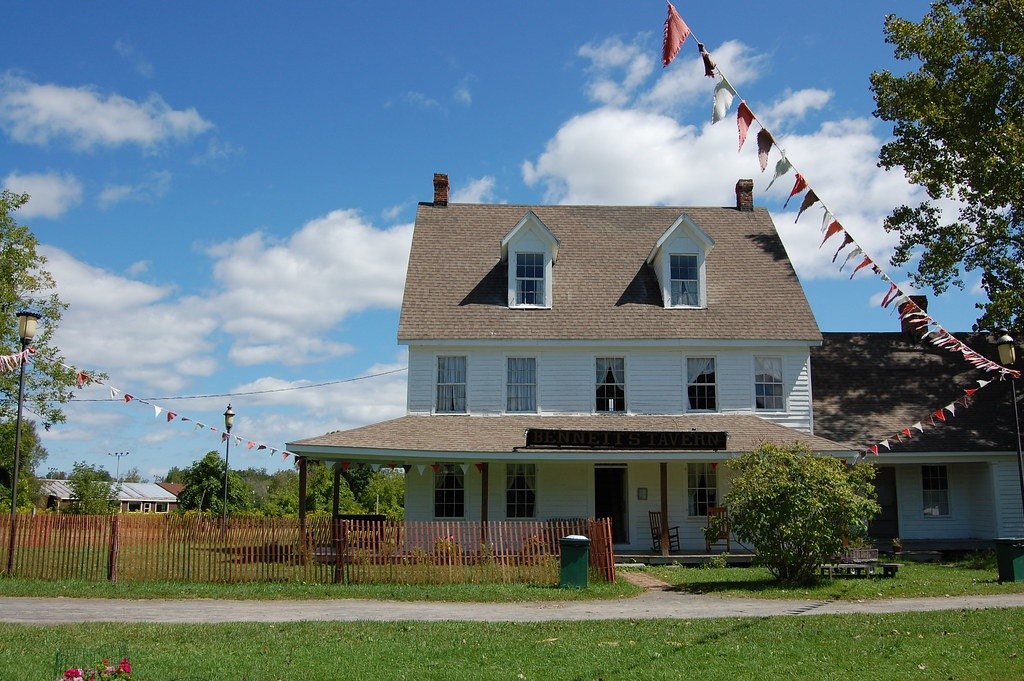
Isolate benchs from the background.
[793,564,904,579]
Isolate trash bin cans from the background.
[558,535,591,588]
[992,537,1024,581]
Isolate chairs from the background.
[704,506,730,553]
[648,510,682,555]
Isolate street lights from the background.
[6,301,42,575]
[223,403,234,539]
[109,451,130,482]
[995,327,1023,508]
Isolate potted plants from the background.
[892,538,903,553]
